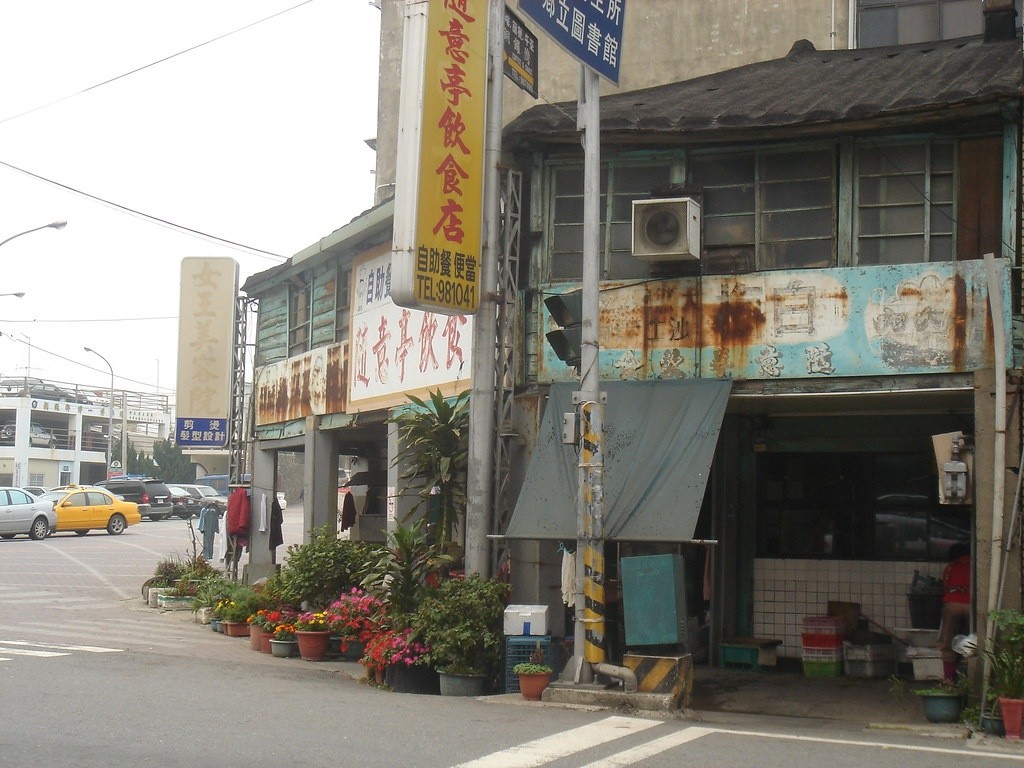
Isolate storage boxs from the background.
[506,635,559,694]
[504,604,550,635]
[801,594,950,680]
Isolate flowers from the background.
[245,588,431,670]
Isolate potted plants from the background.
[918,686,962,723]
[419,571,510,698]
[981,711,1003,736]
[962,637,1024,740]
[514,663,552,701]
[142,524,251,637]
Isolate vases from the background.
[249,624,426,694]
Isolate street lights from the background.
[83,346,113,481]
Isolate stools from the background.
[723,638,782,674]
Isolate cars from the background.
[18,383,110,405]
[0,484,140,541]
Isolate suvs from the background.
[95,477,228,521]
[0,420,57,449]
